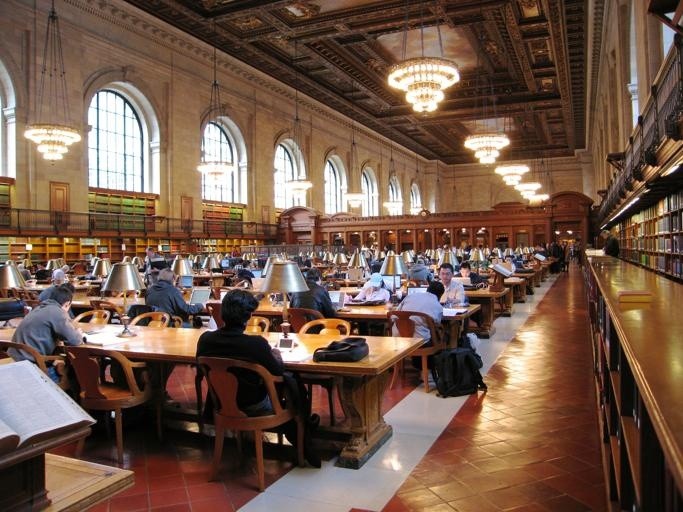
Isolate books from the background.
[0,360,96,458]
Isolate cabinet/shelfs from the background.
[581,257,683,512]
[612,192,683,280]
[1,236,264,263]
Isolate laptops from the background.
[327,291,345,311]
[250,270,262,278]
[219,259,229,270]
[189,288,211,309]
[177,276,192,292]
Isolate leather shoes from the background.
[292,412,320,447]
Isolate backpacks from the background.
[431,347,488,396]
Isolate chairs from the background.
[1,247,535,491]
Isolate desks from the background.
[41,452,135,512]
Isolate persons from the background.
[290,270,336,334]
[392,282,445,382]
[439,263,465,342]
[4,261,74,318]
[7,286,83,382]
[196,289,320,445]
[302,256,311,268]
[356,252,435,336]
[238,260,255,285]
[546,241,577,274]
[144,248,206,328]
[456,244,546,326]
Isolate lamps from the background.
[382,72,402,215]
[387,1,460,117]
[408,138,424,214]
[340,56,369,211]
[465,47,550,206]
[278,36,317,204]
[196,15,242,189]
[23,1,82,164]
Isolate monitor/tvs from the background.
[380,275,394,294]
[394,275,401,289]
[408,287,427,296]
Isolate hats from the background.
[368,272,383,287]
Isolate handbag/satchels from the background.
[312,338,369,362]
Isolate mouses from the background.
[341,308,351,312]
